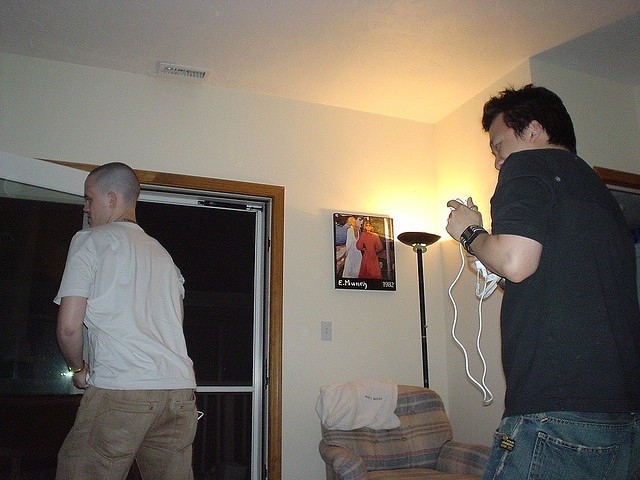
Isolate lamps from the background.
[396,230,443,393]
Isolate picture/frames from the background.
[331,213,397,292]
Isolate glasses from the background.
[366,225,371,227]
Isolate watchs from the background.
[459,226,489,255]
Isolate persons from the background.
[447,84,636,479]
[54,162,198,479]
[342,217,384,279]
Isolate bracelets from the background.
[67,360,86,373]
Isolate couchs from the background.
[314,385,492,479]
